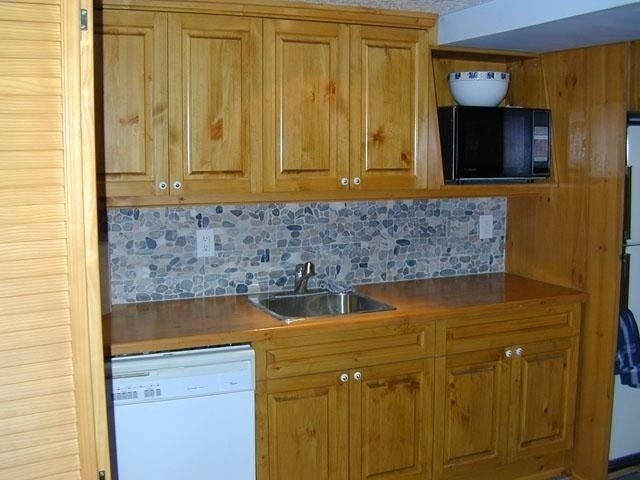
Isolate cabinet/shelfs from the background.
[0,1,113,479]
[254,310,438,480]
[439,293,588,480]
[259,1,439,201]
[95,2,262,206]
[427,46,558,198]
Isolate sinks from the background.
[248,288,397,324]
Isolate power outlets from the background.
[479,215,491,236]
[196,230,214,256]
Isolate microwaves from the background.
[437,105,555,185]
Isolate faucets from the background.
[294,261,316,292]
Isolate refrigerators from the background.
[603,117,640,474]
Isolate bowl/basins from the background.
[448,69,512,108]
